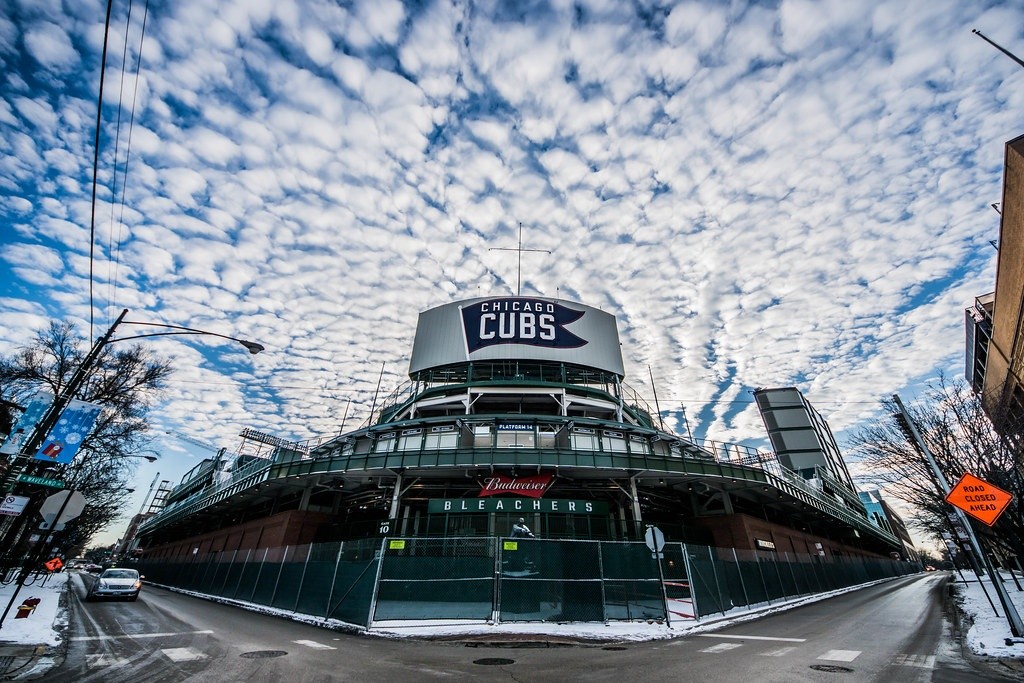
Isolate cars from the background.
[68,563,102,573]
[90,569,146,602]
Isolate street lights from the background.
[0,309,264,506]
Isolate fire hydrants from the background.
[14,598,41,619]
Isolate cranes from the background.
[165,430,237,460]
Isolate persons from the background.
[510,517,535,568]
